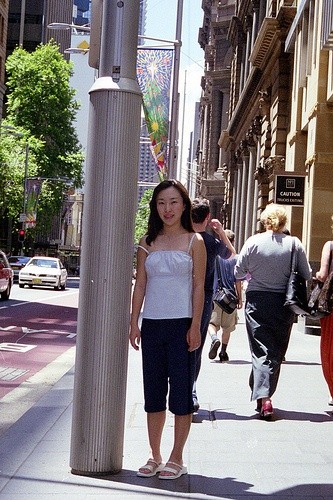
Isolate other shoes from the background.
[193,398,200,411]
[208,339,221,359]
[257,399,262,411]
[219,353,229,362]
[328,401,333,405]
[260,404,274,417]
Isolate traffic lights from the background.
[18,229,25,242]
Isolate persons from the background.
[315,241,333,405]
[233,203,312,418]
[129,179,206,480]
[208,229,243,362]
[191,204,236,411]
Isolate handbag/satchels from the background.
[307,241,333,315]
[213,288,239,314]
[283,237,320,321]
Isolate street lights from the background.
[46,0,185,180]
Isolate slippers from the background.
[136,459,165,477]
[159,462,188,479]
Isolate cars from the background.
[7,256,32,284]
[18,256,68,291]
[0,250,14,301]
